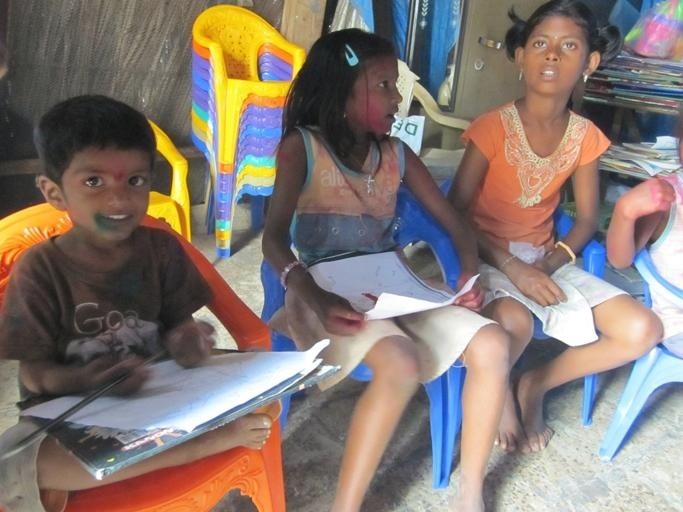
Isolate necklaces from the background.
[348,140,381,198]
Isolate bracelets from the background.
[499,255,517,271]
[280,261,308,288]
[553,241,576,266]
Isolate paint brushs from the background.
[0,349,167,460]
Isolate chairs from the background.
[432,177,605,426]
[398,73,473,180]
[140,116,193,239]
[1,201,288,510]
[187,3,306,257]
[596,246,682,462]
[256,183,455,491]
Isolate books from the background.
[581,51,682,181]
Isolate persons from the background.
[0,93,273,512]
[606,111,683,359]
[259,27,513,511]
[446,1,664,455]
